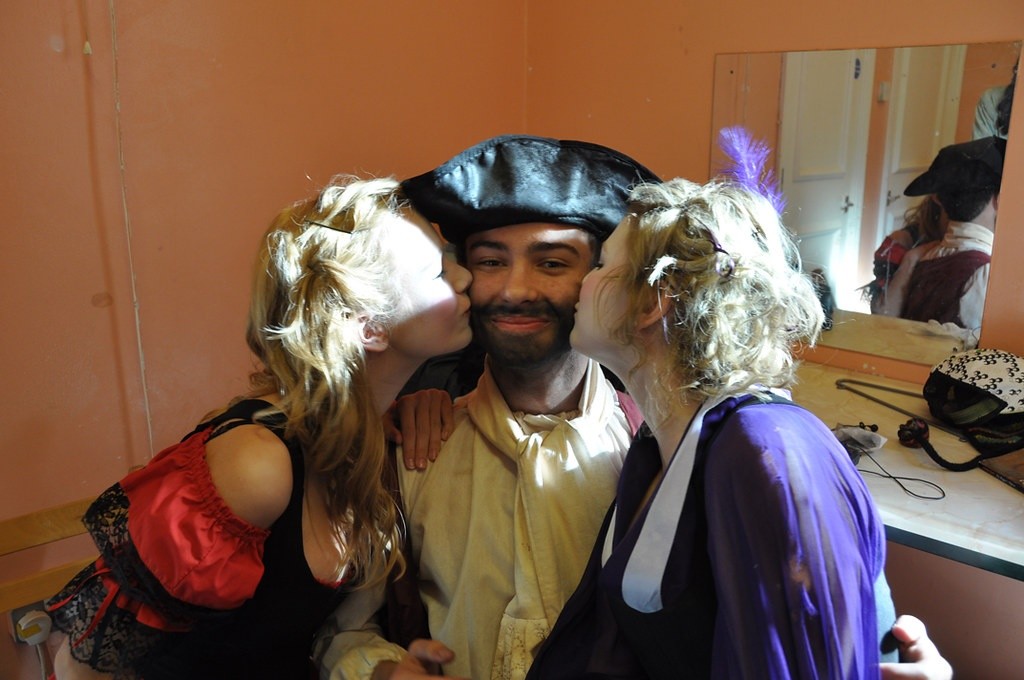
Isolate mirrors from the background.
[710,41,1023,367]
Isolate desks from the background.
[795,359,1024,680]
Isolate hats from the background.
[400,136,663,245]
[903,136,1008,198]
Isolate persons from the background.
[863,58,1019,329]
[50,127,957,680]
